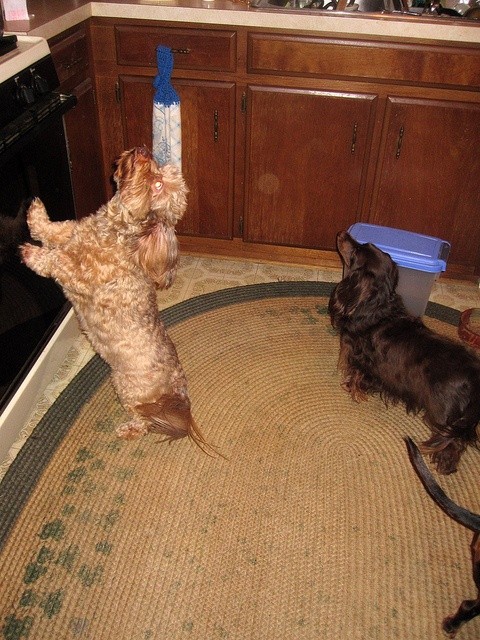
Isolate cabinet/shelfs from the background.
[50,30,108,220]
[109,26,236,242]
[243,33,479,282]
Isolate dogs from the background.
[327,230,479,475]
[17,147,225,462]
[398,434,480,636]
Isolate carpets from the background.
[0,282,480,636]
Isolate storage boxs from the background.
[345,222,450,319]
